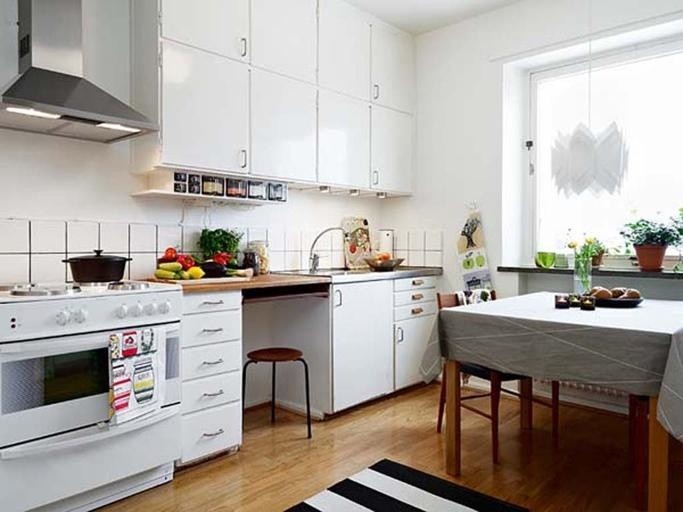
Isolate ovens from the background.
[0,292,181,511]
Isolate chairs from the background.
[436,291,560,464]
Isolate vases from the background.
[573,257,592,295]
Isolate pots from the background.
[61,251,132,282]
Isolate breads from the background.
[590,286,640,299]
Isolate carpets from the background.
[283,459,532,512]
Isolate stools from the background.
[242,347,312,439]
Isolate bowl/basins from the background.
[534,252,556,268]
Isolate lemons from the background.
[189,267,205,278]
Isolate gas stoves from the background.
[0,279,182,305]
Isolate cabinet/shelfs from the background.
[130,1,318,83]
[394,276,440,392]
[130,41,317,182]
[332,280,394,413]
[317,1,416,116]
[180,293,242,463]
[316,89,413,199]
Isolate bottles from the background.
[251,240,269,274]
[243,249,258,275]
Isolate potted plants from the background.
[620,219,682,271]
[582,238,605,266]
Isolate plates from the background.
[593,298,643,307]
[364,259,404,270]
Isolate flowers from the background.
[567,228,605,292]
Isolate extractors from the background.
[0,1,159,144]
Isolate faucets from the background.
[308,227,349,272]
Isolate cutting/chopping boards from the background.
[343,217,371,270]
[147,277,250,285]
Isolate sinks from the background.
[270,269,354,276]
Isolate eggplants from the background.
[198,262,253,278]
[154,262,190,279]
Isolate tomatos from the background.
[165,248,200,266]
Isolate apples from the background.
[377,253,391,260]
